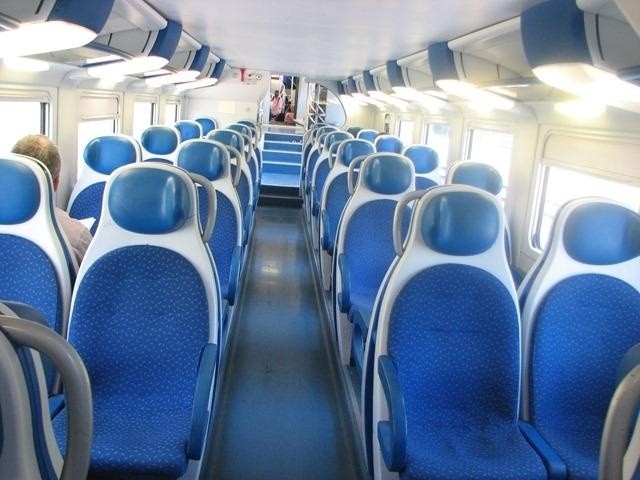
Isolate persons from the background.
[11,135,92,263]
[270,90,283,116]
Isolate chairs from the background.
[373,135,404,156]
[327,153,378,341]
[140,126,180,167]
[351,184,464,476]
[237,121,262,189]
[1,333,50,480]
[355,128,380,142]
[66,132,143,238]
[598,344,640,480]
[362,182,568,480]
[0,301,94,480]
[348,127,362,138]
[299,128,318,196]
[403,144,442,190]
[446,162,526,311]
[47,162,222,478]
[167,162,229,430]
[205,129,253,247]
[229,126,259,214]
[197,119,217,139]
[320,139,380,292]
[301,123,328,145]
[517,196,603,313]
[175,121,201,141]
[175,138,243,351]
[18,155,78,289]
[0,153,71,420]
[333,152,416,378]
[305,126,339,223]
[307,131,355,251]
[519,197,640,480]
